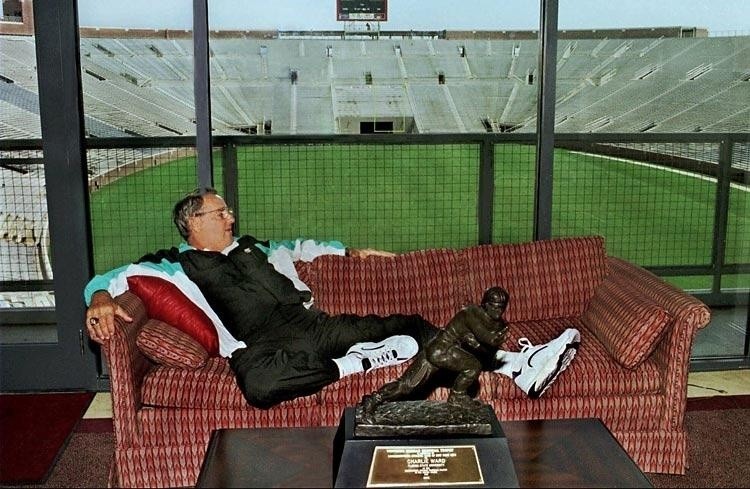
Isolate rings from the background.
[90,317,100,326]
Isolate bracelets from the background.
[345,247,350,257]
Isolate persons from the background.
[82,185,583,412]
[353,286,513,424]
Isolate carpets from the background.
[37,391,750,489]
[0,390,95,489]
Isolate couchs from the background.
[100,231,713,489]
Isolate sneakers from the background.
[509,328,581,400]
[345,334,419,374]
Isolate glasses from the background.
[195,206,234,219]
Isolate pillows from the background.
[580,268,678,374]
[133,313,215,374]
[125,272,226,357]
